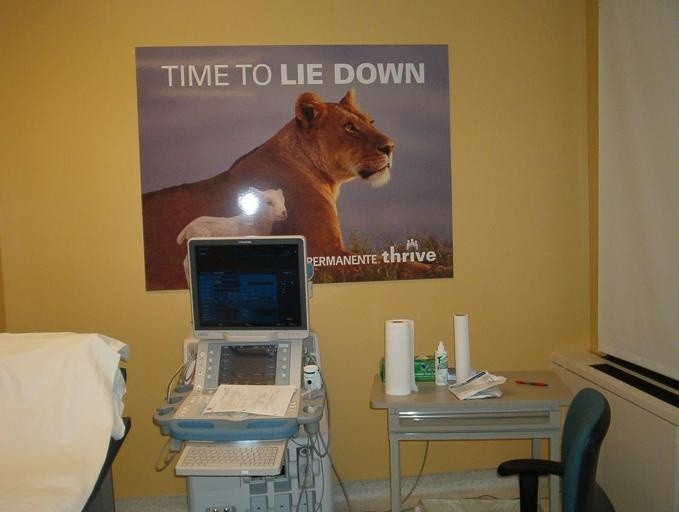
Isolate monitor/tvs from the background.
[187,237,309,339]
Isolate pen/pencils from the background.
[515,381,547,386]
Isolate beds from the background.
[1,330,132,511]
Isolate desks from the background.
[370,371,574,511]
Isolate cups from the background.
[303,365,323,391]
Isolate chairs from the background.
[414,384,614,511]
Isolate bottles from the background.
[435,341,449,386]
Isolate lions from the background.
[141,88,452,291]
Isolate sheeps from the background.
[176,186,287,288]
